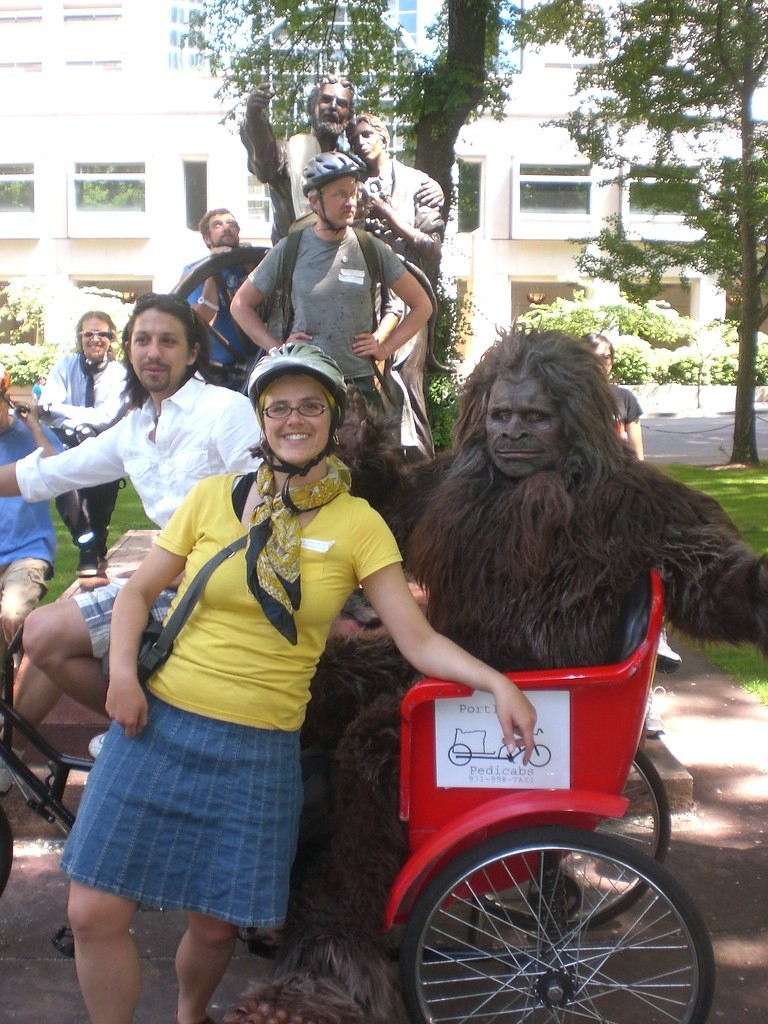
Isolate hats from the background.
[36,376,41,381]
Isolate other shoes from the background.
[75,548,99,576]
[0,767,14,795]
[87,731,109,759]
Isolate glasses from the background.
[135,292,195,324]
[262,401,327,419]
[328,191,357,200]
[78,331,108,337]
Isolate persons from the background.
[0,293,537,1024]
[230,152,433,410]
[580,332,644,461]
[239,76,457,466]
[178,207,273,367]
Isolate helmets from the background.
[247,341,348,410]
[0,365,11,395]
[301,151,360,198]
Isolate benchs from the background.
[348,568,663,933]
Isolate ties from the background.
[79,350,110,408]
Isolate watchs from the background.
[198,297,219,311]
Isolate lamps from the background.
[118,285,136,304]
[524,286,549,305]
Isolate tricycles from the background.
[0,566,715,1024]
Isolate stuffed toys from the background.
[237,322,768,1024]
